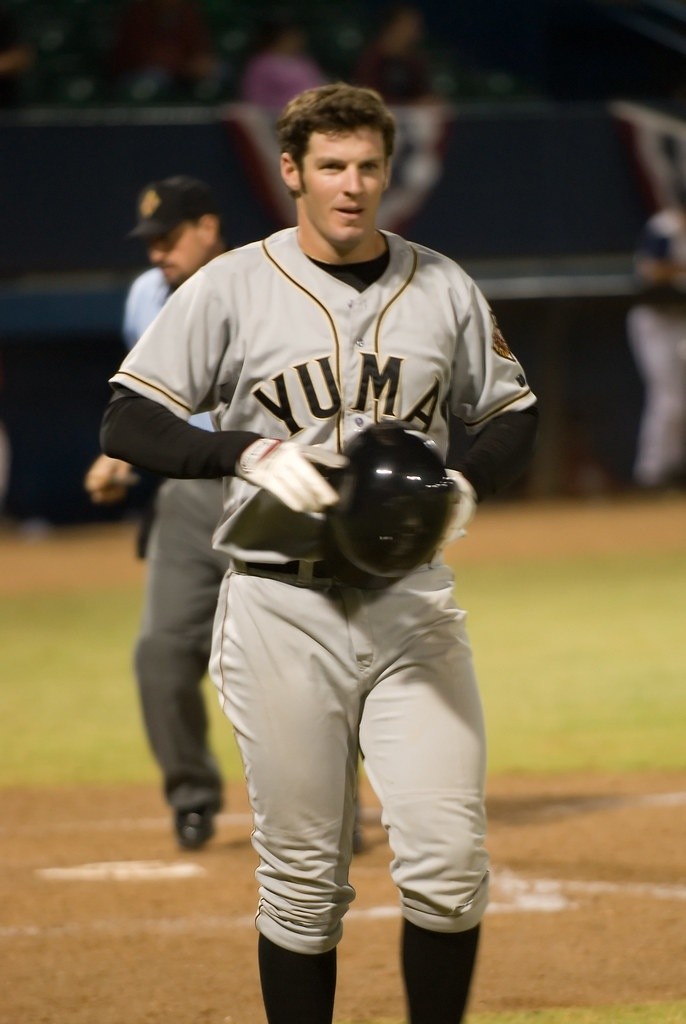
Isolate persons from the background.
[101,84,536,1024]
[80,179,235,845]
[623,186,686,487]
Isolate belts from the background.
[230,560,331,585]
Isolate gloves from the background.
[441,468,477,546]
[235,437,350,518]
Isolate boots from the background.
[258,932,337,1024]
[400,919,480,1024]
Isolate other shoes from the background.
[176,809,213,847]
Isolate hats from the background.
[124,171,220,237]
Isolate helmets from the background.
[328,418,456,579]
